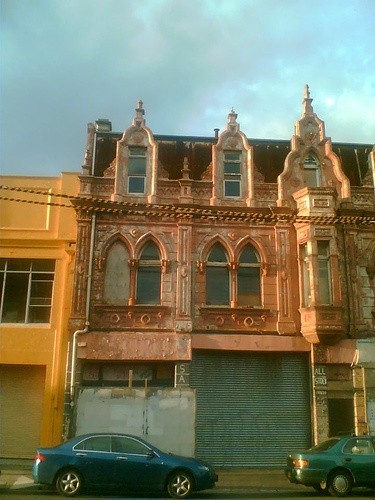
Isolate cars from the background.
[32,433,217,499]
[283,434,375,497]
